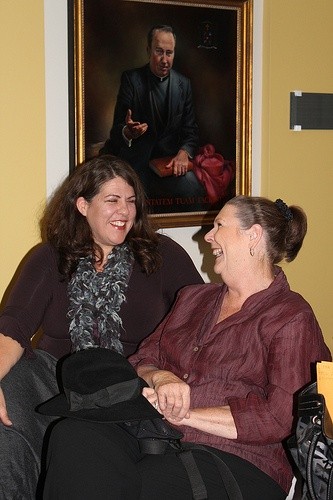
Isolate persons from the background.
[0,157,205,500]
[41,195,333,500]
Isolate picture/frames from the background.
[67,0,253,228]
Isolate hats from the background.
[33,347,165,424]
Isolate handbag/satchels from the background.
[280,380,333,500]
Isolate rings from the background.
[153,400,158,409]
